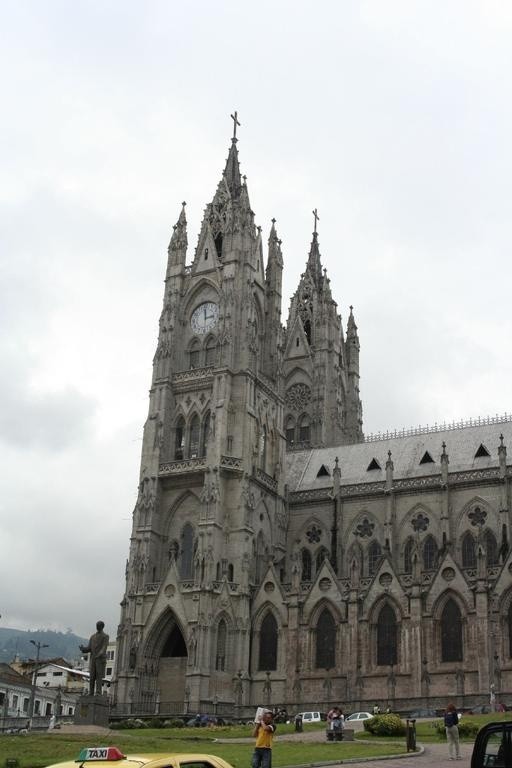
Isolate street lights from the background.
[25,638,51,718]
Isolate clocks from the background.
[188,300,221,336]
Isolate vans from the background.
[285,710,327,725]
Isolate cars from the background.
[343,712,375,721]
[460,703,509,715]
[40,744,235,768]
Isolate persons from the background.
[332,710,345,742]
[202,712,212,728]
[194,711,201,728]
[249,712,278,768]
[327,707,337,741]
[444,704,465,760]
[386,704,392,714]
[79,620,110,697]
[372,704,380,714]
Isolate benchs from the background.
[326,728,354,740]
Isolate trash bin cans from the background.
[407,719,416,752]
[7,757,19,768]
[296,716,303,733]
